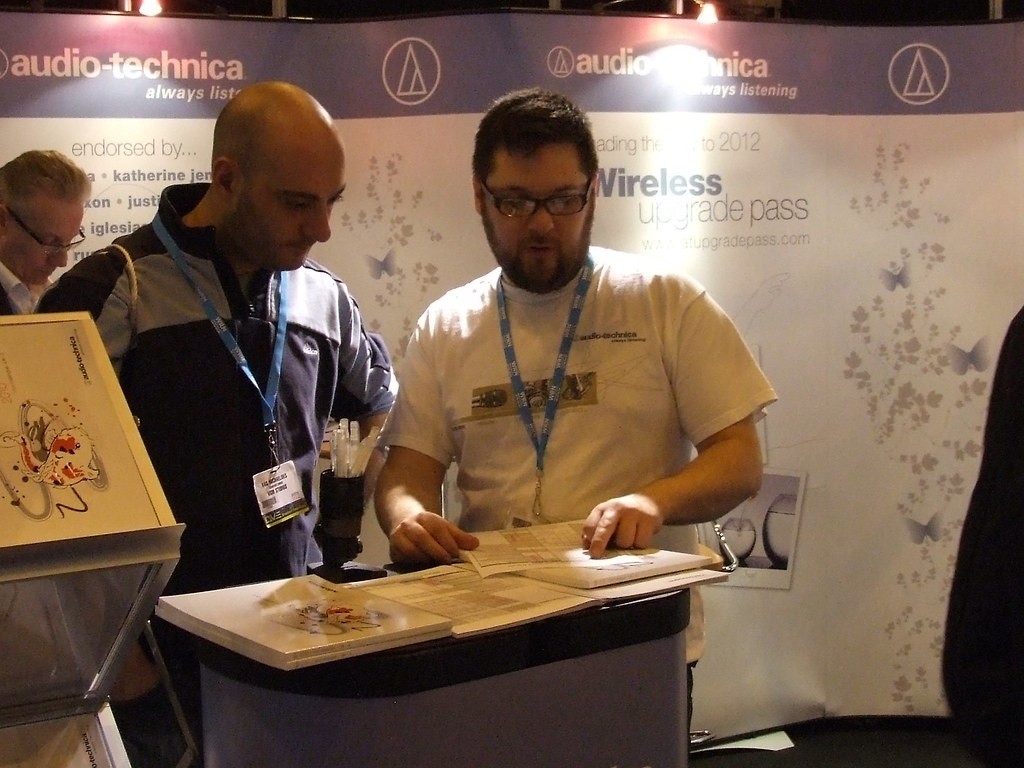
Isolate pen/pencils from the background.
[330,418,380,478]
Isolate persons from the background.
[943,305,1024,768]
[0,150,90,317]
[374,89,777,752]
[33,79,400,768]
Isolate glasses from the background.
[4,205,85,257]
[477,176,595,219]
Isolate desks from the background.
[152,566,726,768]
